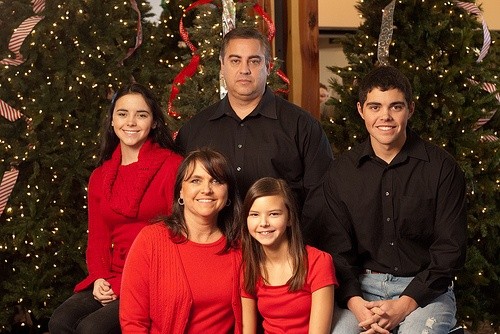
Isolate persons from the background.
[49,83,184,334]
[172,26,334,224]
[322,66,468,334]
[240,178,339,334]
[119,148,242,334]
